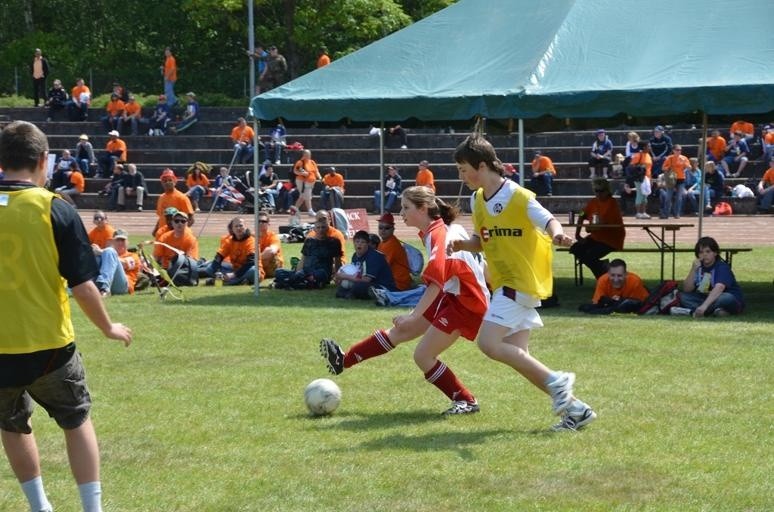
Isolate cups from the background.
[591,214,599,223]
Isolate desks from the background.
[561,224,695,284]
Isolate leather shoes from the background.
[100,291,111,296]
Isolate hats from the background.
[160,170,177,180]
[80,135,88,140]
[164,207,188,218]
[113,230,127,240]
[377,214,394,224]
[108,129,119,136]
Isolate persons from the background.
[232,116,288,165]
[415,159,436,195]
[0,119,133,512]
[530,148,558,198]
[369,122,408,148]
[33,46,90,117]
[446,131,598,434]
[248,46,331,97]
[589,258,650,313]
[677,236,745,319]
[162,47,179,108]
[568,120,774,219]
[48,132,149,213]
[568,177,626,281]
[88,210,412,299]
[319,185,494,415]
[152,172,195,235]
[374,166,404,213]
[182,148,345,217]
[107,83,199,136]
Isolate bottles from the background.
[214,271,223,288]
[287,156,290,163]
[671,306,696,317]
[568,210,575,224]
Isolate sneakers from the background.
[715,309,731,316]
[442,400,480,415]
[117,206,143,211]
[309,209,317,217]
[547,373,597,431]
[320,339,344,374]
[636,212,651,219]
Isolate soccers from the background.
[304,379,341,414]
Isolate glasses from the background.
[379,226,392,229]
[175,220,186,224]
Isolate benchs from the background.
[556,248,752,288]
